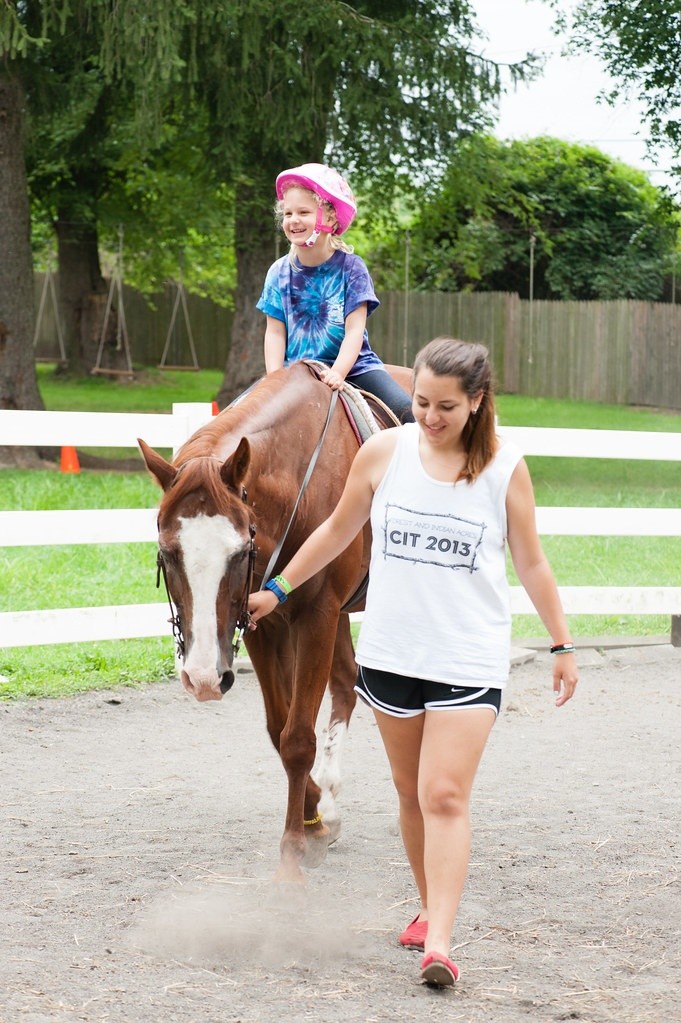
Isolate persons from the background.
[256,163,417,426]
[249,337,579,986]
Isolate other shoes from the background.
[420,953,458,984]
[400,912,429,951]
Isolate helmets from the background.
[276,162,358,235]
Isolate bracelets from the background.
[263,578,287,604]
[550,641,576,654]
[275,575,292,593]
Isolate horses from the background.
[136,359,416,885]
